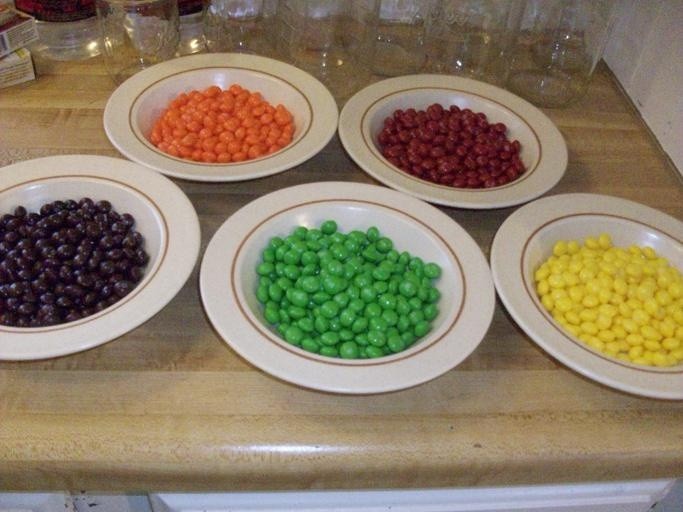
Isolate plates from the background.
[2,151,202,364]
[339,72,569,209]
[489,190,680,402]
[206,180,498,397]
[101,52,340,183]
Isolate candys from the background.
[378,102,526,188]
[536,235,682,365]
[255,220,442,359]
[150,83,295,164]
[0,197,151,327]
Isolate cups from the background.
[95,1,623,108]
